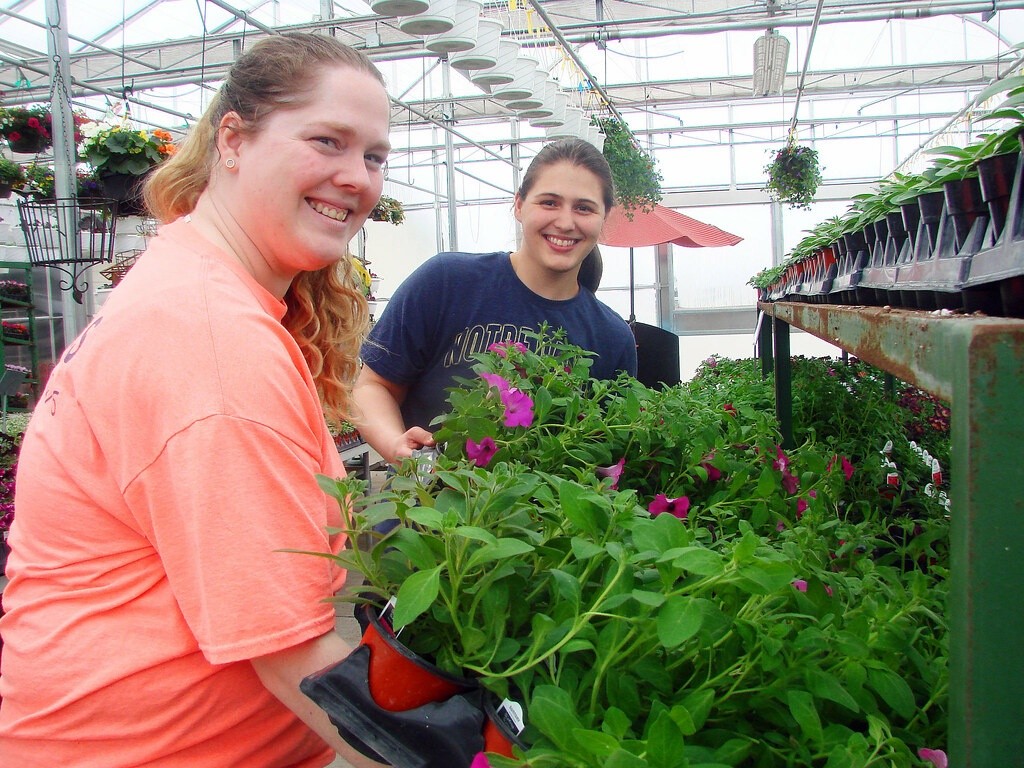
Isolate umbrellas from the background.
[598,190,742,248]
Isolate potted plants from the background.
[746,41,1024,303]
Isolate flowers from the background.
[12,162,53,196]
[0,279,29,294]
[5,364,31,374]
[0,106,88,156]
[76,168,97,194]
[2,321,30,334]
[9,392,31,403]
[596,112,664,222]
[0,410,34,542]
[760,143,826,211]
[81,126,177,175]
[0,158,27,184]
[275,321,1024,768]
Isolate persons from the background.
[1,29,398,768]
[353,138,640,477]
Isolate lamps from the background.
[752,30,791,98]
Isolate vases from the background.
[580,117,594,142]
[3,333,29,346]
[1,295,28,310]
[506,70,549,109]
[397,0,457,35]
[7,133,42,153]
[545,108,584,141]
[517,81,558,119]
[424,0,483,53]
[359,602,461,712]
[35,192,56,204]
[0,180,12,198]
[8,401,27,407]
[0,544,8,573]
[101,174,148,218]
[530,92,567,128]
[492,56,539,99]
[77,192,103,210]
[595,134,607,153]
[368,0,430,16]
[447,17,504,70]
[588,126,599,145]
